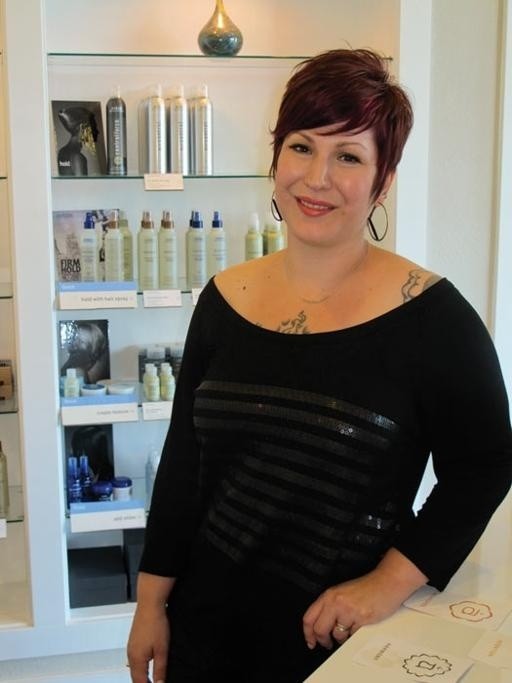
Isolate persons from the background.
[123,47,511,682]
[57,106,100,177]
[61,322,108,384]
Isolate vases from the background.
[196,0,242,56]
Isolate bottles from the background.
[144,448,161,498]
[97,475,133,503]
[65,455,94,510]
[65,367,81,403]
[140,340,184,402]
[142,83,216,177]
[80,211,227,289]
[242,209,284,265]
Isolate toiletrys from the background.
[80,212,98,282]
[185,212,206,290]
[106,211,134,283]
[206,209,226,277]
[246,213,283,258]
[148,83,211,175]
[159,210,178,291]
[65,455,134,504]
[136,211,157,290]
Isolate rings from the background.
[335,621,349,631]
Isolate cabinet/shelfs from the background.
[0,174,36,631]
[50,47,432,613]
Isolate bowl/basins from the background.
[81,379,138,396]
